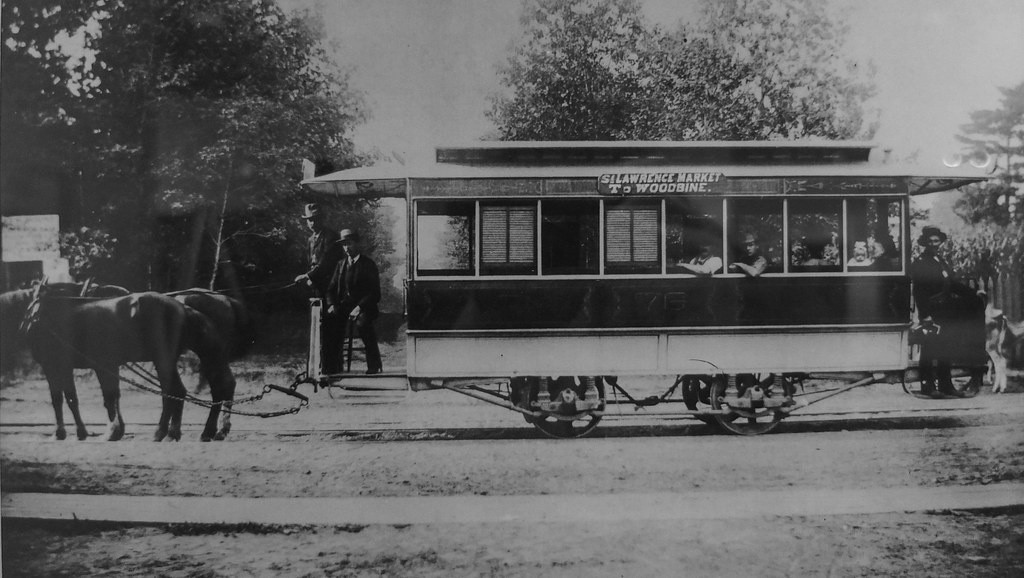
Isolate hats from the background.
[301,204,322,219]
[917,225,946,246]
[336,229,359,243]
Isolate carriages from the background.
[0,136,1000,442]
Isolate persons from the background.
[294,201,341,298]
[675,238,723,274]
[913,223,960,401]
[729,232,767,277]
[849,241,873,266]
[326,230,383,375]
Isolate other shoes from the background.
[366,365,383,374]
[922,389,945,399]
[940,387,965,397]
[322,365,343,375]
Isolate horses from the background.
[0,276,253,442]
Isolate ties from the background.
[351,258,354,265]
